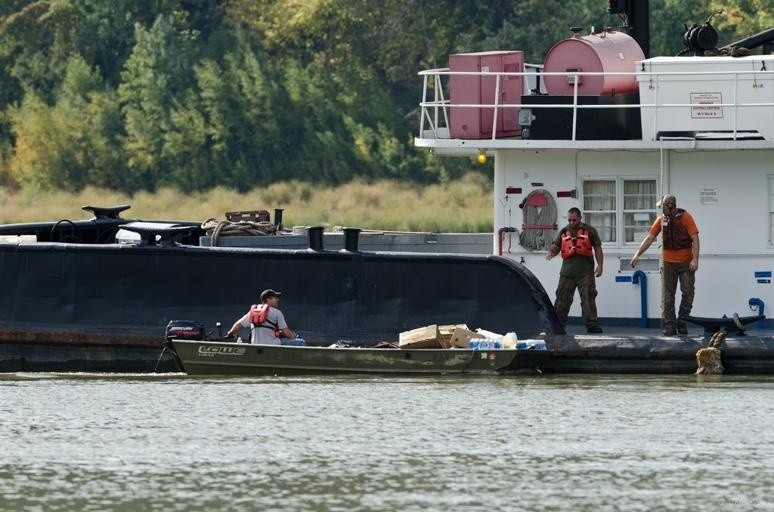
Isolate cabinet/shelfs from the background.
[448,50,524,139]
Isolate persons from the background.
[547,207,603,333]
[226,289,305,345]
[631,194,699,336]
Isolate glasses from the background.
[569,219,576,221]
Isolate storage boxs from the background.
[398,323,486,349]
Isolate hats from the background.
[260,289,282,300]
[656,194,676,208]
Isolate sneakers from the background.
[587,325,602,333]
[664,321,688,335]
